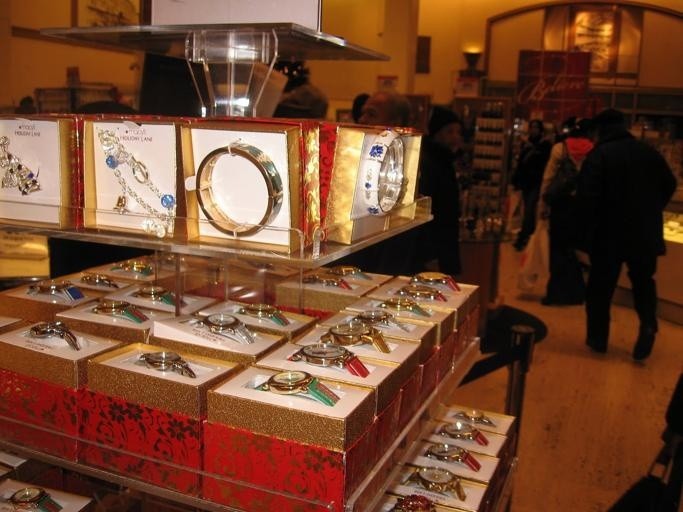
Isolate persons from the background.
[273,84,462,277]
[508,108,676,360]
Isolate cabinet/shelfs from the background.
[0,0,519,512]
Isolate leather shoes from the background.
[584,338,609,353]
[632,324,656,361]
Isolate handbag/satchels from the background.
[511,220,551,300]
[605,477,666,511]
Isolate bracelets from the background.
[198,143,283,237]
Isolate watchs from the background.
[1,254,497,511]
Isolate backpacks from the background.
[543,142,580,219]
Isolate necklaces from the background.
[0,130,175,227]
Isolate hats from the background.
[427,104,461,135]
